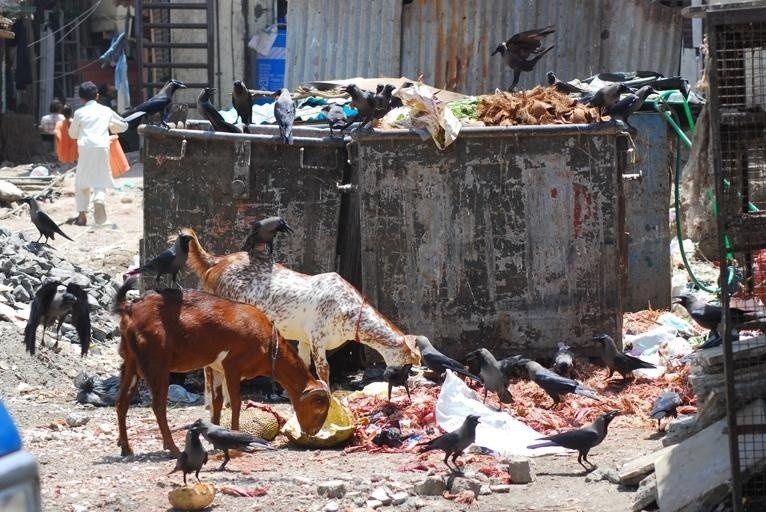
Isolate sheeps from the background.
[111,275,332,459]
[167,227,423,421]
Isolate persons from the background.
[41,98,67,135]
[64,104,74,128]
[68,80,129,227]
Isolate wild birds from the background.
[180,418,278,472]
[646,391,682,433]
[717,264,739,297]
[130,233,196,289]
[16,196,75,244]
[239,215,294,261]
[526,409,621,472]
[414,413,483,475]
[23,279,91,358]
[490,25,557,93]
[545,69,660,130]
[382,333,658,412]
[167,426,209,486]
[119,77,414,146]
[670,292,766,339]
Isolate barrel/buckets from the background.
[111,133,130,179]
[257,19,286,104]
